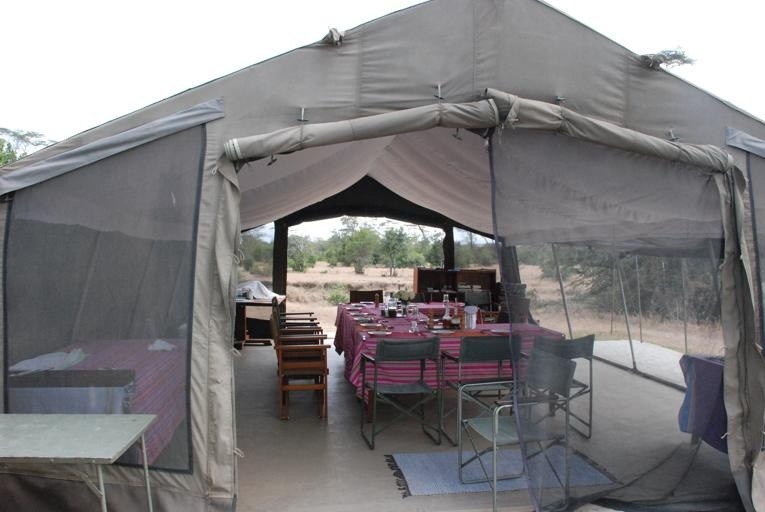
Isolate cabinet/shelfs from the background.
[414,267,498,295]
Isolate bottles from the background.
[441,298,461,330]
[380,293,404,317]
[237,288,254,300]
[375,294,379,308]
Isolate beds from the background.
[8,335,188,467]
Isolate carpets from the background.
[382,440,625,499]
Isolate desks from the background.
[0,412,162,512]
[234,294,288,350]
[677,351,727,456]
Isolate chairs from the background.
[436,336,531,448]
[359,336,442,451]
[269,288,532,420]
[457,348,577,512]
[517,333,597,439]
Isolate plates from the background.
[346,300,374,317]
[355,317,392,337]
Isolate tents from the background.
[0,0,765,512]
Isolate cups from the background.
[443,294,449,305]
[464,307,478,330]
[409,322,417,333]
[406,303,420,320]
